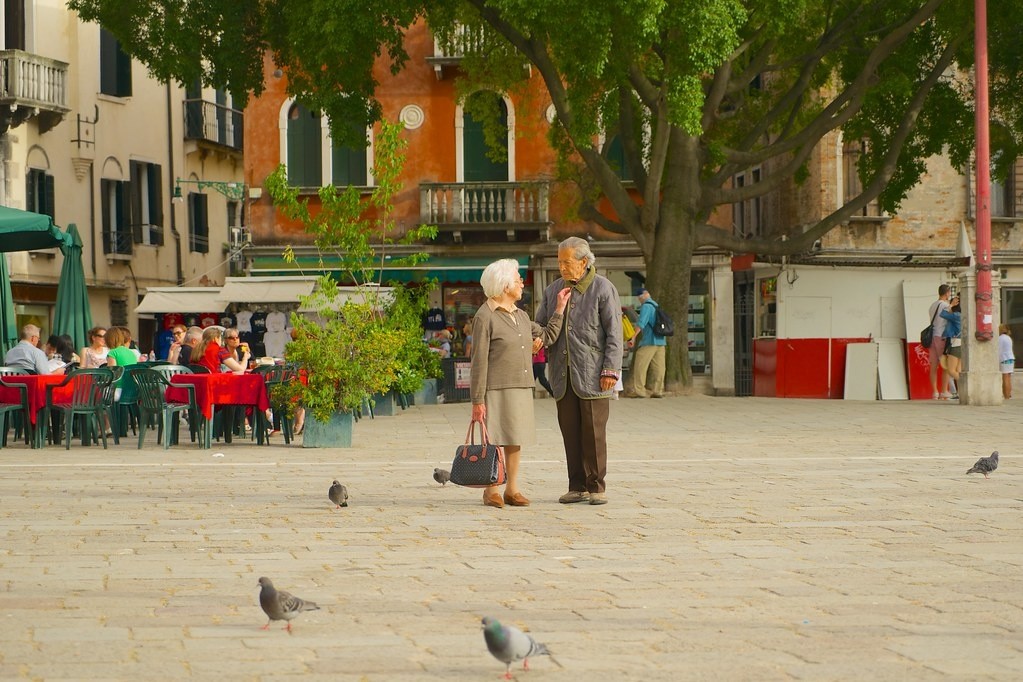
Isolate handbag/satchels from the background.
[449,419,507,488]
[921,325,933,348]
[943,337,952,355]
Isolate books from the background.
[48,358,80,373]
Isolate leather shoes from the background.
[483,490,504,507]
[503,490,531,506]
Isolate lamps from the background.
[171,177,246,203]
[451,288,460,295]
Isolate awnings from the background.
[134,292,230,313]
[216,282,316,303]
[296,291,397,313]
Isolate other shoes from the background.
[932,392,939,400]
[627,391,645,398]
[948,393,959,400]
[650,393,662,398]
[609,393,619,400]
[939,392,953,401]
[559,491,590,503]
[590,493,608,505]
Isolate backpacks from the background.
[642,302,674,337]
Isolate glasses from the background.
[32,335,41,341]
[225,337,238,340]
[173,331,185,335]
[514,278,523,284]
[94,334,104,338]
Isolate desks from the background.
[225,368,339,432]
[0,375,102,446]
[164,374,270,450]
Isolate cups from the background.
[249,360,256,369]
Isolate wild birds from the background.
[253,577,322,632]
[433,467,451,488]
[480,615,549,679]
[328,480,350,509]
[965,451,1000,479]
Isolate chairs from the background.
[0,361,412,449]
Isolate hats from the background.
[637,288,647,295]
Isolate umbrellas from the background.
[0,205,94,366]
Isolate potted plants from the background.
[262,120,446,449]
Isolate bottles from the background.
[149,348,156,362]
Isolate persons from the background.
[158,325,297,437]
[532,237,624,505]
[463,323,473,358]
[621,289,667,398]
[610,307,635,400]
[998,324,1015,400]
[532,345,554,399]
[80,326,142,439]
[471,258,572,508]
[4,324,67,375]
[292,328,314,436]
[44,334,80,439]
[928,285,962,401]
[436,330,450,359]
